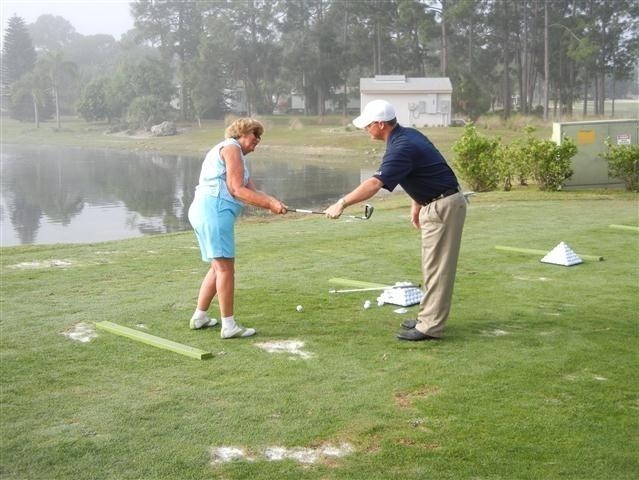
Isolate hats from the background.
[353,101,396,128]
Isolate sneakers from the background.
[220,323,254,339]
[189,317,216,329]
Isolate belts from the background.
[422,188,458,206]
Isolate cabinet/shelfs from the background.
[548,118,639,191]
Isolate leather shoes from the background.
[398,329,434,340]
[402,319,418,328]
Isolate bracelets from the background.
[338,198,346,207]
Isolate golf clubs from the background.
[285,205,374,220]
[328,285,421,293]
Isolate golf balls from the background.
[376,281,423,306]
[364,301,371,309]
[541,242,582,265]
[296,306,303,311]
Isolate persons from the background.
[324,99,467,340]
[185,117,290,339]
